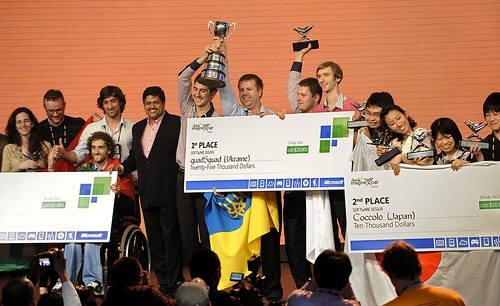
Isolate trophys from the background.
[292,25,320,51]
[366,129,402,167]
[346,100,369,129]
[406,130,434,160]
[198,21,236,88]
[460,120,489,149]
[21,151,42,172]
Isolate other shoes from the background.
[52,279,63,294]
[87,281,105,298]
[39,286,49,295]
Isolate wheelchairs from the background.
[101,194,151,279]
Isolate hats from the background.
[174,281,209,306]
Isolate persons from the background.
[0,106,54,260]
[107,86,184,298]
[351,90,500,176]
[286,249,361,306]
[175,44,219,249]
[0,249,82,306]
[214,36,283,306]
[282,42,361,291]
[381,241,466,306]
[51,86,141,296]
[37,89,102,149]
[99,246,239,306]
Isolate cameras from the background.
[32,251,55,271]
[230,272,244,282]
